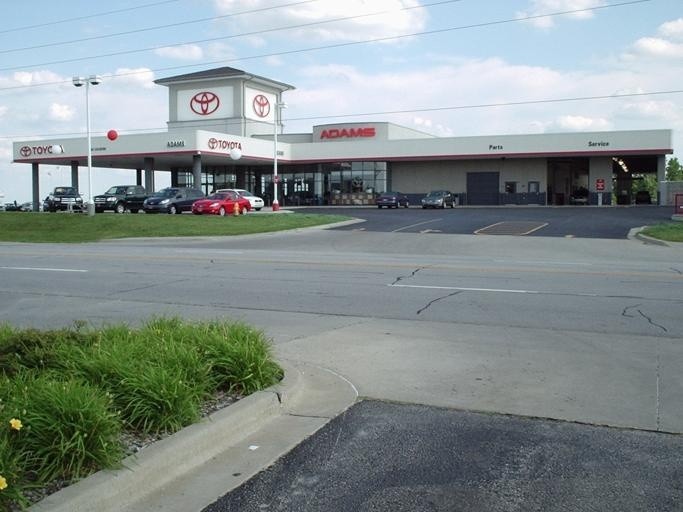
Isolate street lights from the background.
[272,101,288,211]
[73,75,102,216]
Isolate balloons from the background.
[51,146,62,156]
[107,130,117,141]
[229,147,242,161]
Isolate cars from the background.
[93,185,264,216]
[636,191,651,204]
[421,191,456,209]
[570,191,588,205]
[43,187,86,213]
[376,192,409,209]
